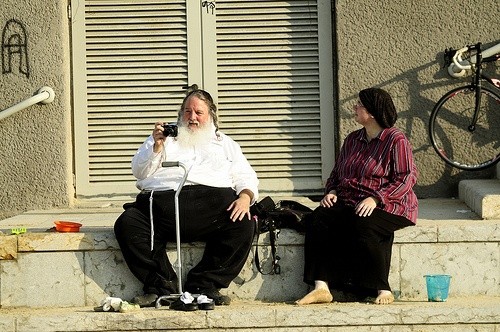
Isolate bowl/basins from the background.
[54,221,82,231]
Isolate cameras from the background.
[160,122,178,137]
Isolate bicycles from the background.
[428,40,499,172]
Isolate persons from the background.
[294,88,419,305]
[114,89,260,308]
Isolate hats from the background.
[359,88,398,130]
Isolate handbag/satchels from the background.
[250,196,281,275]
[261,199,313,235]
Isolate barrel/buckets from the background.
[423,274,452,302]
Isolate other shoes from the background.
[130,293,158,306]
[205,290,231,306]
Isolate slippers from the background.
[170,296,198,311]
[197,303,215,310]
